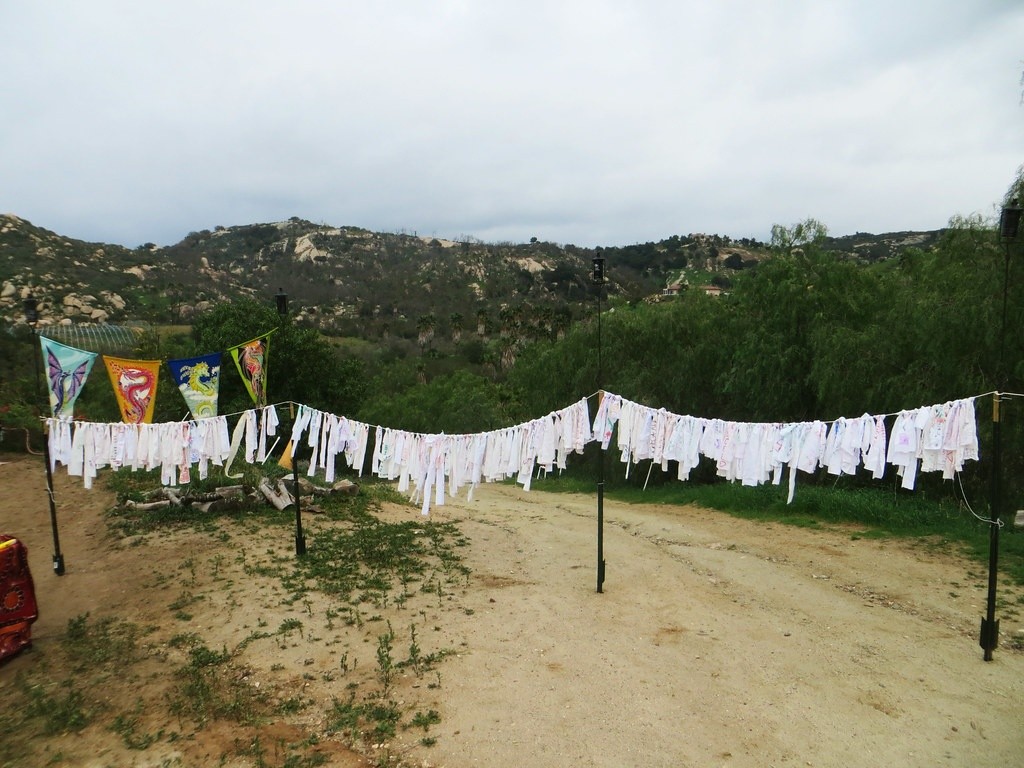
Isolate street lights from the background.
[976,202,1024,661]
[272,287,306,557]
[592,251,606,594]
[21,292,66,576]
